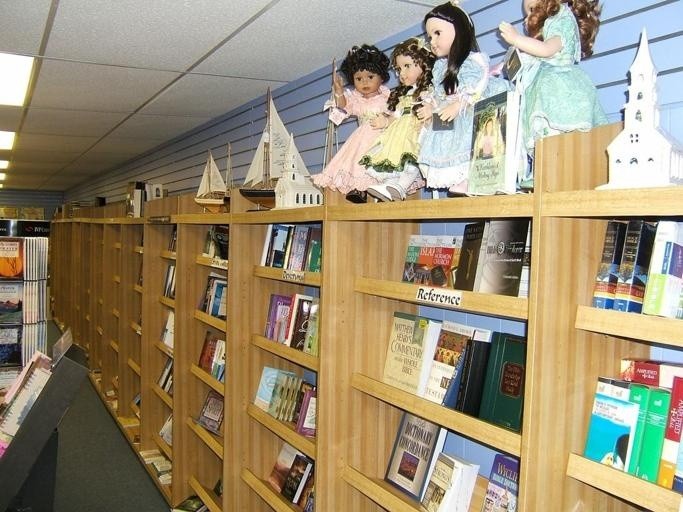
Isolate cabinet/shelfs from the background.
[230,185,323,508]
[324,157,531,508]
[524,119,682,508]
[176,192,230,508]
[48,194,176,506]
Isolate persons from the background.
[310,0,491,203]
[498,0,607,194]
[309,0,607,204]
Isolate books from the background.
[383,312,527,433]
[401,218,532,298]
[130,392,140,418]
[384,413,519,512]
[0,217,73,464]
[133,434,223,512]
[255,365,317,512]
[128,224,230,350]
[155,331,226,437]
[260,223,324,359]
[583,219,683,495]
